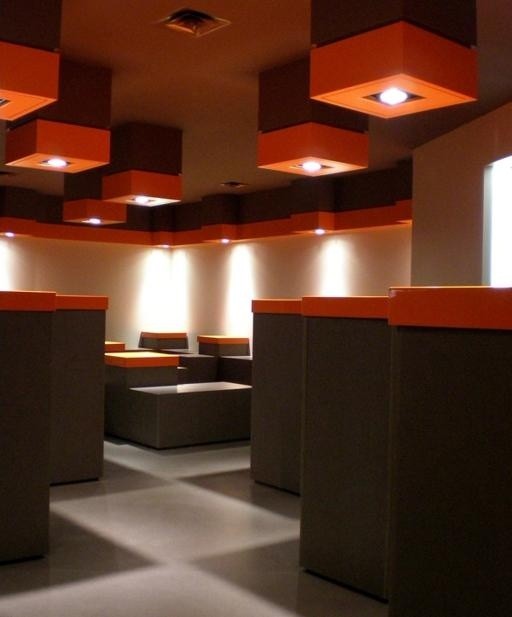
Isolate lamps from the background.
[308,1,478,120]
[5,64,111,173]
[1,218,27,238]
[102,124,181,207]
[63,169,127,226]
[256,62,369,178]
[291,179,335,235]
[0,1,60,122]
[205,193,235,244]
